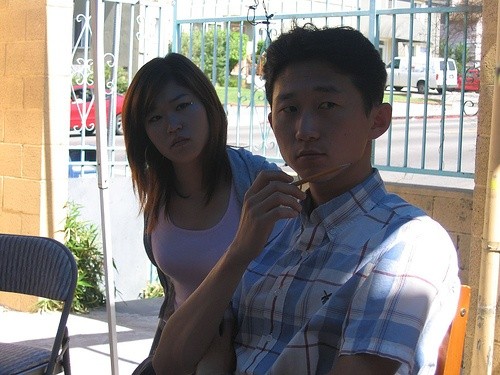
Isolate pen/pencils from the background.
[289,163,352,187]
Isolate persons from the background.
[72,92,83,104]
[153,23,461,375]
[122,52,279,375]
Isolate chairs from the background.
[437,284,472,375]
[0,233,78,375]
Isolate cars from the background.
[70,84,126,135]
[456,69,480,92]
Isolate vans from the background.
[384,57,458,94]
